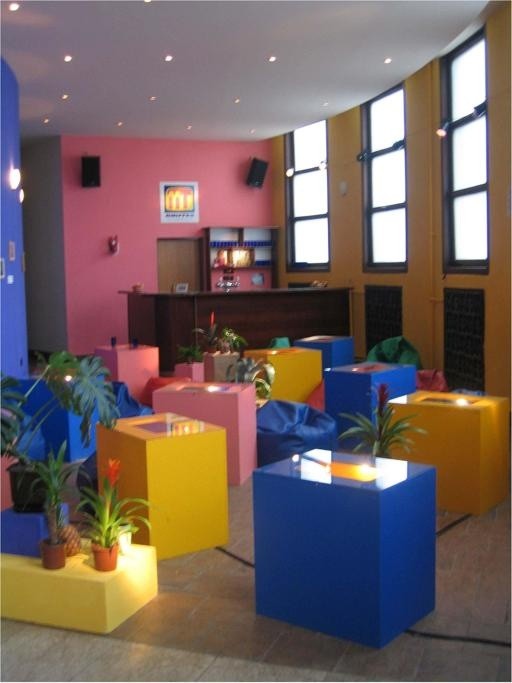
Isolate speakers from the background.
[246,157,269,188]
[81,155,101,187]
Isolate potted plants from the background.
[70,456,160,573]
[28,437,89,570]
[0,347,123,514]
[176,308,250,364]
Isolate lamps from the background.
[435,119,450,137]
[474,99,488,118]
